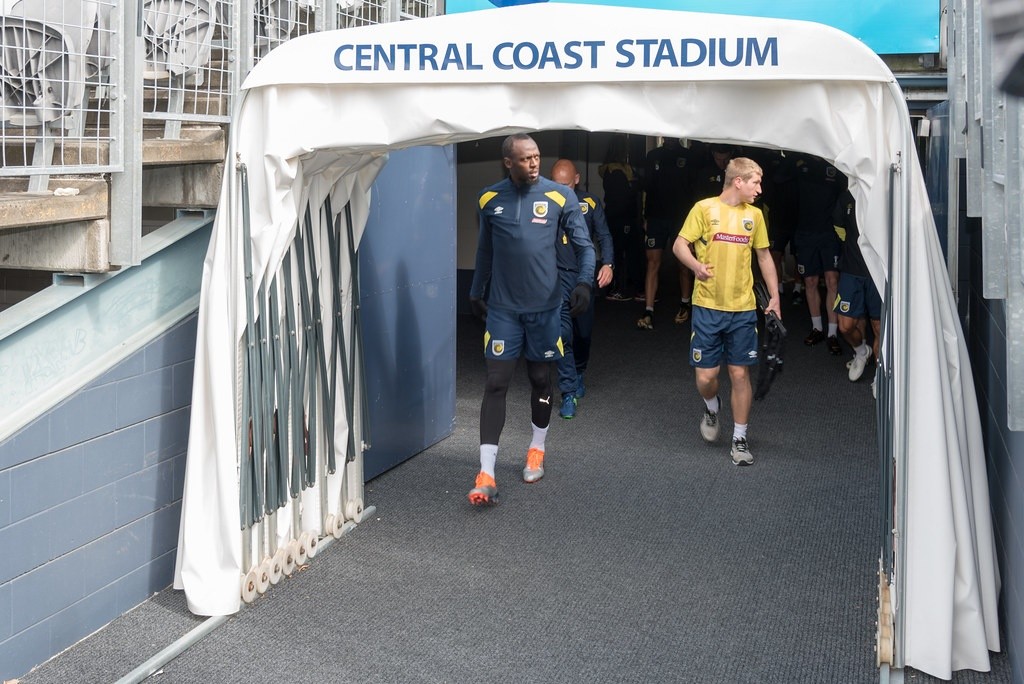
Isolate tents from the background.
[171,3,1002,682]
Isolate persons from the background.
[551,158,615,421]
[467,133,596,506]
[672,157,782,467]
[598,134,883,401]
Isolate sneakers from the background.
[638,310,655,330]
[803,329,824,345]
[870,369,878,399]
[699,396,722,443]
[826,334,842,355]
[848,345,872,381]
[845,354,867,369]
[730,435,754,465]
[523,447,544,481]
[561,394,577,419]
[573,374,585,399]
[675,301,690,323]
[468,472,499,506]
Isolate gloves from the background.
[569,285,591,316]
[470,300,489,328]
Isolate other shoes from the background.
[605,290,632,301]
[634,294,658,302]
